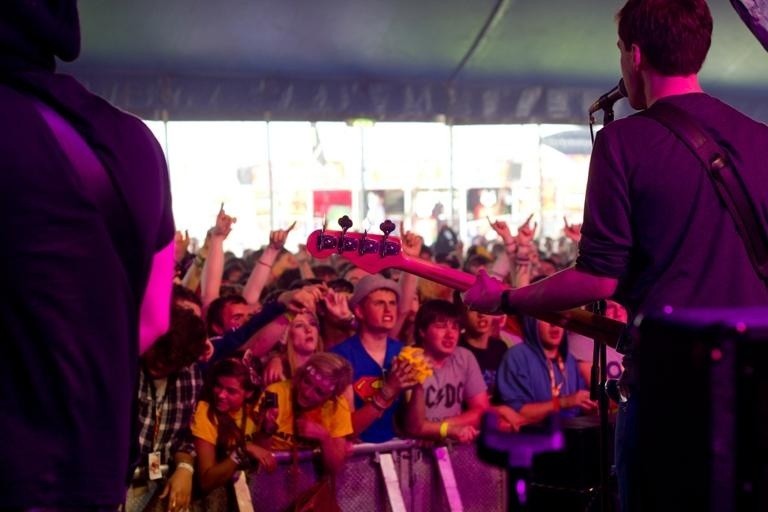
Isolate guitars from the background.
[307,214,639,404]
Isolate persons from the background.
[469,1,767,512]
[3,1,212,511]
[121,204,627,511]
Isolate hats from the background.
[467,245,490,264]
[347,274,401,313]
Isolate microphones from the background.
[587,77,628,113]
